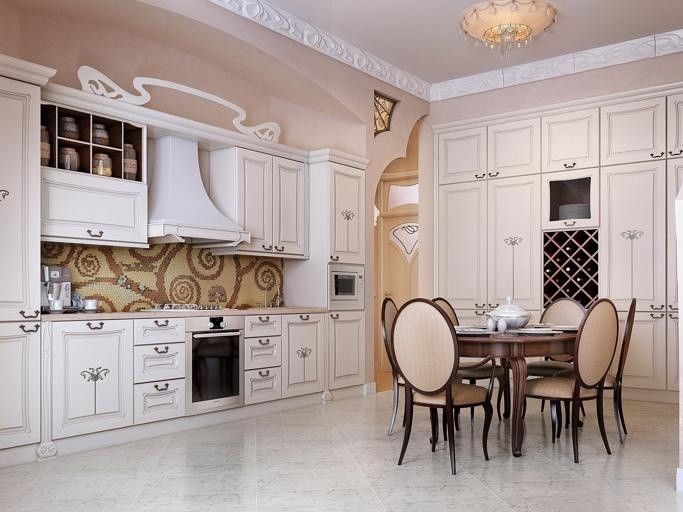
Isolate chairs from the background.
[518,298,619,464]
[390,298,496,475]
[380,295,636,444]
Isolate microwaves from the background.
[330,271,358,301]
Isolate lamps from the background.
[460,0,559,57]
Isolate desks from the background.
[429,326,584,458]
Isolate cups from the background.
[45,299,65,310]
[83,299,101,308]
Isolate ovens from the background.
[184,329,244,413]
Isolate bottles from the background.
[58,116,79,140]
[40,126,50,167]
[92,153,112,177]
[59,147,79,171]
[91,124,109,146]
[122,143,136,180]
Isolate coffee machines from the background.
[39,265,78,313]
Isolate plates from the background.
[511,323,579,337]
[452,325,499,337]
[79,307,100,313]
[44,309,66,313]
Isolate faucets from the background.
[263,283,280,308]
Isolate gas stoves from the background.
[138,303,238,312]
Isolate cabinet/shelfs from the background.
[431,174,543,392]
[52,319,134,440]
[281,311,328,399]
[329,310,365,390]
[541,166,601,231]
[541,107,600,171]
[0,321,41,451]
[134,316,186,425]
[601,97,683,167]
[41,83,151,249]
[0,75,42,322]
[244,314,281,406]
[436,173,543,309]
[600,157,683,311]
[435,116,541,185]
[453,307,541,380]
[282,160,367,265]
[209,146,310,260]
[540,229,600,312]
